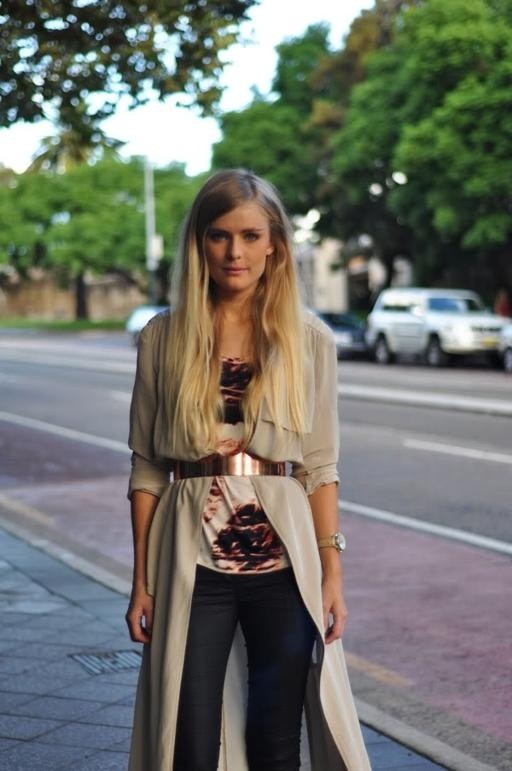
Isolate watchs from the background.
[317,529,348,553]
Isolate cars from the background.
[497,325,512,371]
[306,308,365,361]
[127,307,169,346]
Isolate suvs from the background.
[361,287,512,368]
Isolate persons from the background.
[493,285,511,316]
[121,164,374,769]
